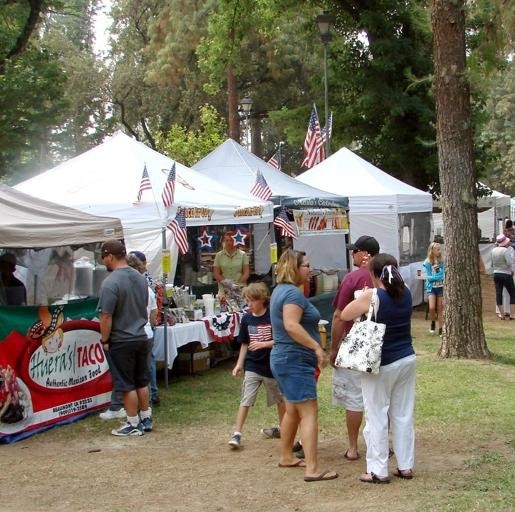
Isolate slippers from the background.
[392,467,414,479]
[278,459,306,468]
[344,447,360,459]
[359,471,391,484]
[304,470,338,481]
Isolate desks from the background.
[145,259,426,390]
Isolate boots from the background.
[430,320,442,338]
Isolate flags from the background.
[160,161,176,208]
[273,204,298,240]
[267,141,282,171]
[301,105,333,169]
[167,209,190,255]
[249,169,273,201]
[132,165,153,204]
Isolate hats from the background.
[95,240,126,255]
[129,251,145,262]
[494,234,510,246]
[345,235,379,253]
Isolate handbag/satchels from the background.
[334,320,387,374]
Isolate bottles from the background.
[417,269,421,276]
[214,294,221,313]
[331,208,348,229]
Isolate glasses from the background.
[301,264,310,267]
[352,249,360,253]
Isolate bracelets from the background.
[99,338,110,345]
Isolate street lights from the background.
[240,90,254,151]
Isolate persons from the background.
[211,229,249,295]
[422,219,515,336]
[128,251,161,408]
[96,253,148,419]
[96,238,154,436]
[269,246,340,482]
[338,253,420,484]
[329,234,395,462]
[228,282,287,448]
[0,252,29,306]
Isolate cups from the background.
[190,295,196,307]
[166,283,173,297]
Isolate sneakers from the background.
[261,427,280,438]
[291,442,304,457]
[228,433,242,448]
[100,408,153,436]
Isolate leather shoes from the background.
[497,313,515,320]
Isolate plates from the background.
[0,377,34,435]
